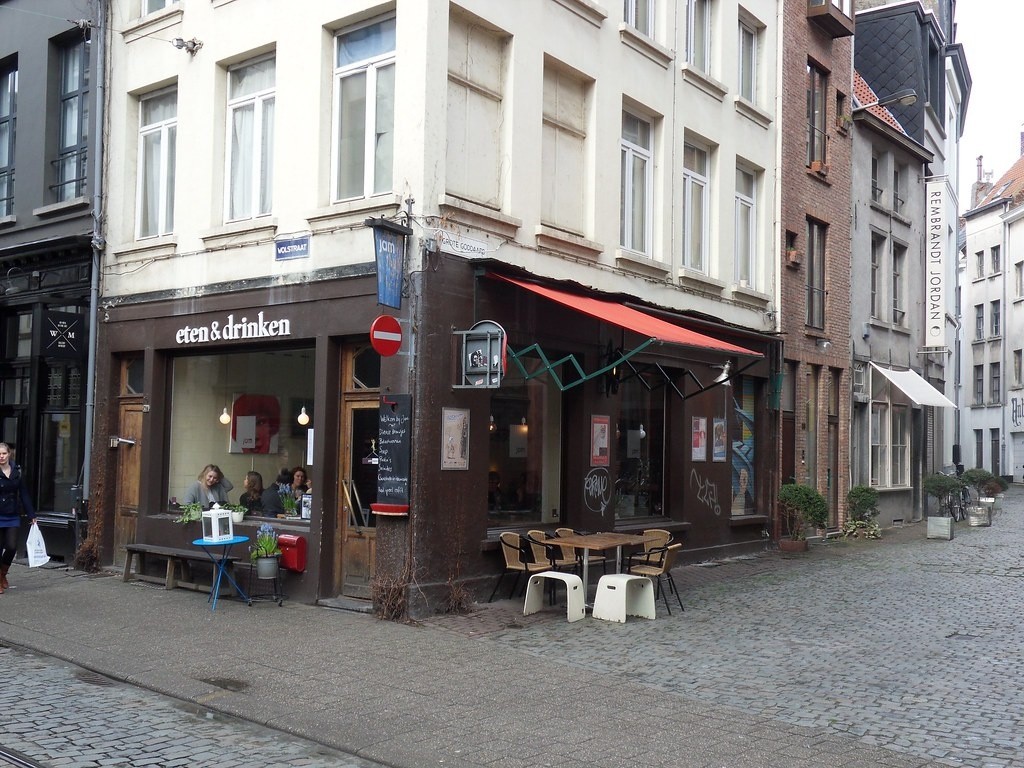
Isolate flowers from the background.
[173,504,201,524]
[251,522,280,558]
[222,503,248,513]
[281,491,299,509]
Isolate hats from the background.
[276,468,294,483]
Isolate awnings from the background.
[869,361,958,410]
[497,272,765,401]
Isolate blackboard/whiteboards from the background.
[376,394,412,506]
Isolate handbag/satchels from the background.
[25,523,50,567]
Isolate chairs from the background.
[626,543,684,615]
[554,528,607,595]
[623,529,674,601]
[528,529,580,605]
[488,531,552,603]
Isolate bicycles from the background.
[938,469,972,521]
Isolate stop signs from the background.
[371,315,404,356]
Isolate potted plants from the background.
[811,159,833,175]
[774,485,829,554]
[989,477,1008,510]
[923,475,959,538]
[840,486,881,539]
[978,483,999,511]
[837,114,853,130]
[958,469,995,527]
[784,246,803,261]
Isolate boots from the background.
[0,565,9,588]
[0,582,4,593]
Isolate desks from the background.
[539,534,658,615]
[193,536,251,610]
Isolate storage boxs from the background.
[202,509,233,541]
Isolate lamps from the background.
[812,338,833,349]
[854,88,917,113]
[5,267,47,296]
[713,361,734,387]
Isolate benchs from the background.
[121,543,240,600]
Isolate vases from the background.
[231,510,243,523]
[258,554,278,579]
[284,507,293,515]
[189,507,202,519]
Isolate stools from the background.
[593,573,655,622]
[523,569,586,623]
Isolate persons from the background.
[261,466,312,518]
[0,443,38,594]
[232,394,281,454]
[488,471,531,511]
[183,464,233,508]
[239,471,265,513]
